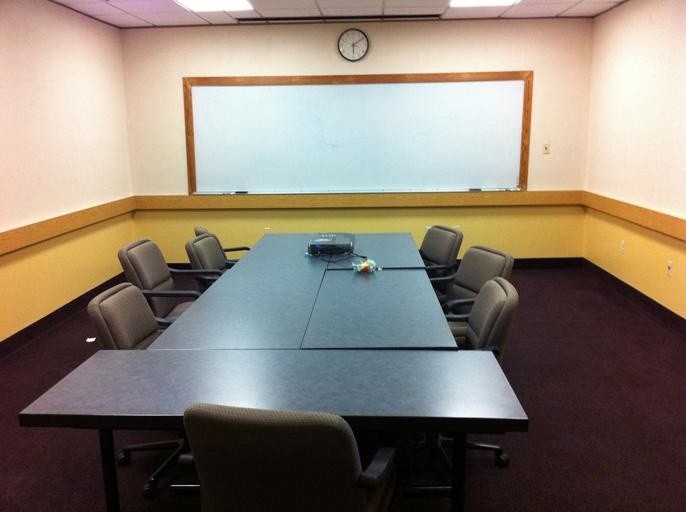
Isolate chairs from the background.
[181,399,412,511]
[86,281,196,490]
[117,240,225,328]
[195,226,250,262]
[429,245,514,349]
[398,275,519,485]
[185,234,237,290]
[417,225,463,305]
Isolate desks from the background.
[328,232,425,269]
[18,351,527,512]
[145,232,333,350]
[301,269,458,352]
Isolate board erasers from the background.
[235,191,248,194]
[469,189,481,191]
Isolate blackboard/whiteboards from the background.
[182,71,533,196]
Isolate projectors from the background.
[307,231,356,256]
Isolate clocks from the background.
[337,28,369,61]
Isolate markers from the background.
[496,189,521,192]
